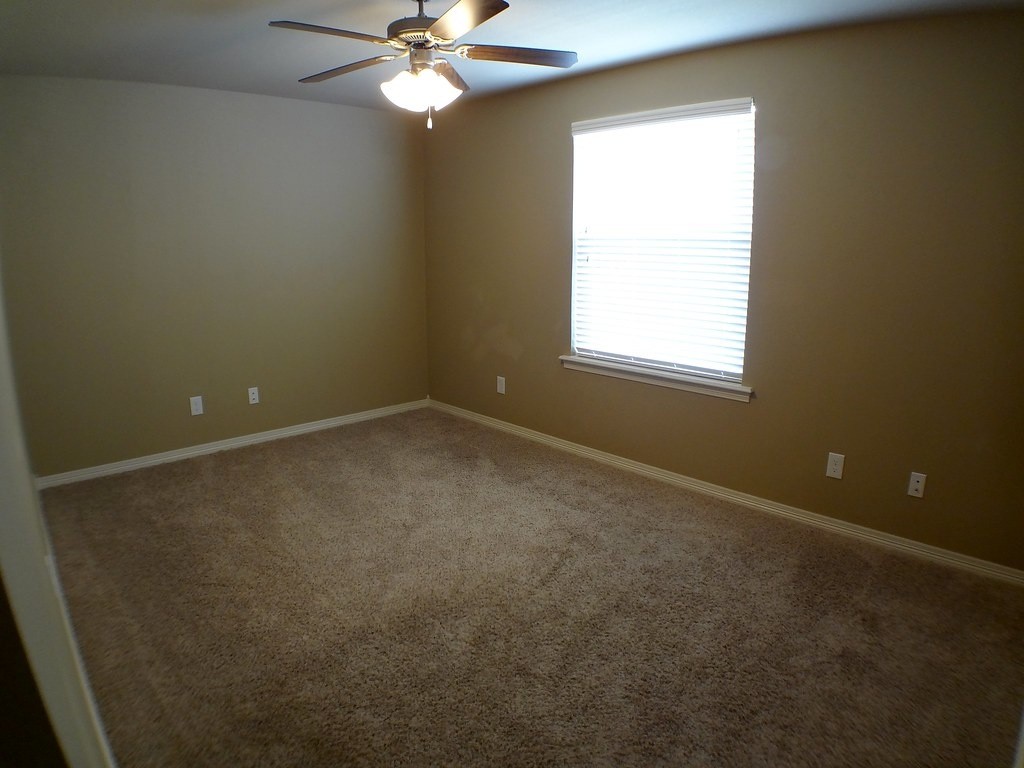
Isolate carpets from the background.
[40,408,1024,768]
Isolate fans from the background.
[269,1,578,84]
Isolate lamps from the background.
[380,49,470,129]
